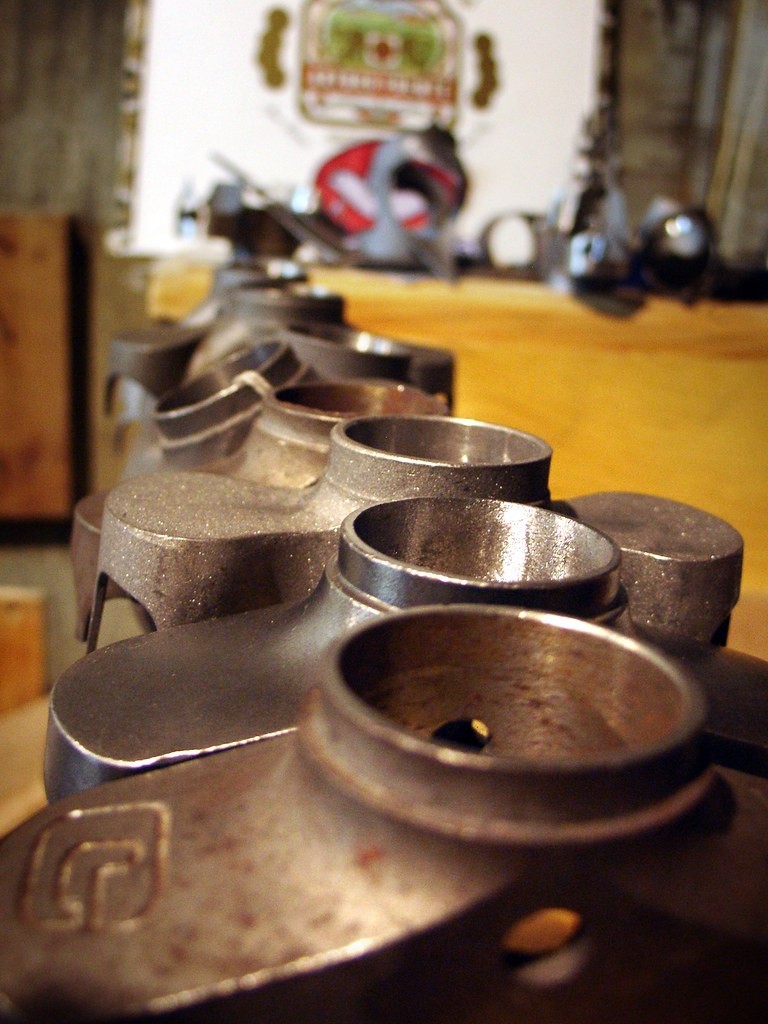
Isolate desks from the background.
[147,257,768,660]
